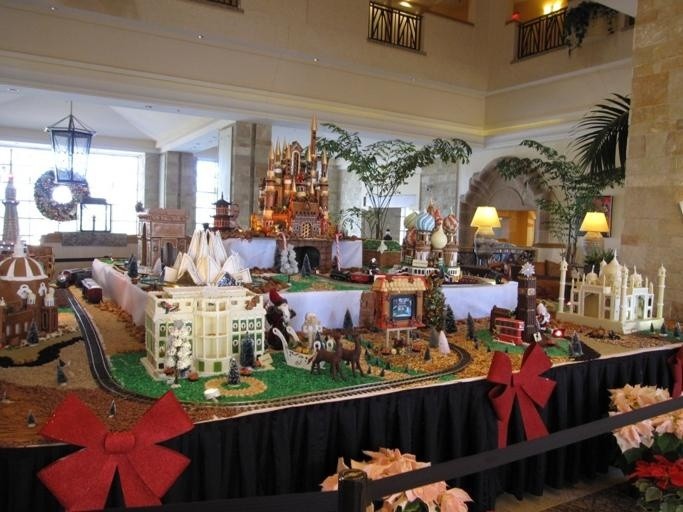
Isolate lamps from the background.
[468,206,501,258]
[578,211,609,257]
[44,99,96,184]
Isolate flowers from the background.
[318,446,474,512]
[606,383,682,512]
